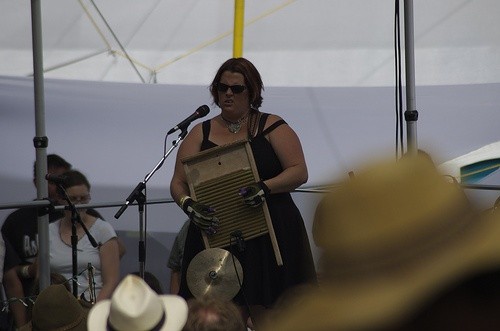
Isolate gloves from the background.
[183,198,220,235]
[237,181,271,208]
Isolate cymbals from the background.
[185,248,245,301]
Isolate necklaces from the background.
[216,111,250,134]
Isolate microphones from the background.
[167,104,210,135]
[46,174,67,182]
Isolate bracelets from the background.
[22,264,33,281]
[177,194,188,207]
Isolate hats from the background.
[86,273,188,331]
[14,283,92,331]
[262,146,499,331]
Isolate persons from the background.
[35,170,120,310]
[87,274,188,331]
[1,155,105,331]
[265,154,500,331]
[31,284,88,331]
[182,292,245,331]
[167,57,316,331]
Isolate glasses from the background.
[69,194,91,204]
[217,83,248,95]
